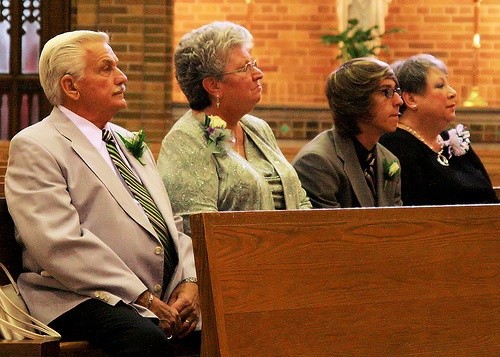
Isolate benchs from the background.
[190,204,500,357]
[0,141,500,357]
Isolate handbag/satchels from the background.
[0,262,61,341]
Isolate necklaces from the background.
[236,129,240,154]
[399,122,443,153]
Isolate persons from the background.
[157,21,314,235]
[292,56,404,208]
[4,30,202,357]
[377,53,498,207]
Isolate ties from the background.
[363,152,377,196]
[102,128,176,290]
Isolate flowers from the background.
[114,129,149,167]
[382,157,400,192]
[194,111,236,157]
[442,124,471,159]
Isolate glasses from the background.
[376,87,404,99]
[212,59,258,77]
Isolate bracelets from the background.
[148,293,153,309]
[181,277,197,283]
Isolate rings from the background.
[167,335,172,340]
[186,320,189,323]
